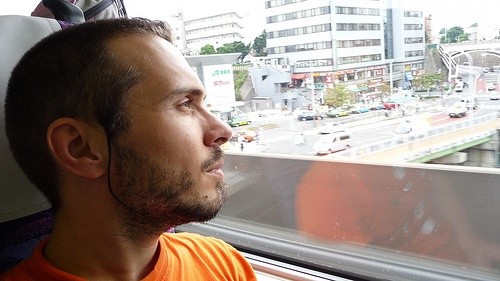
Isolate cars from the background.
[225,62,500,142]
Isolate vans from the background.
[314,133,350,155]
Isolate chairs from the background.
[0,15,175,275]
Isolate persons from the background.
[0,17,258,281]
